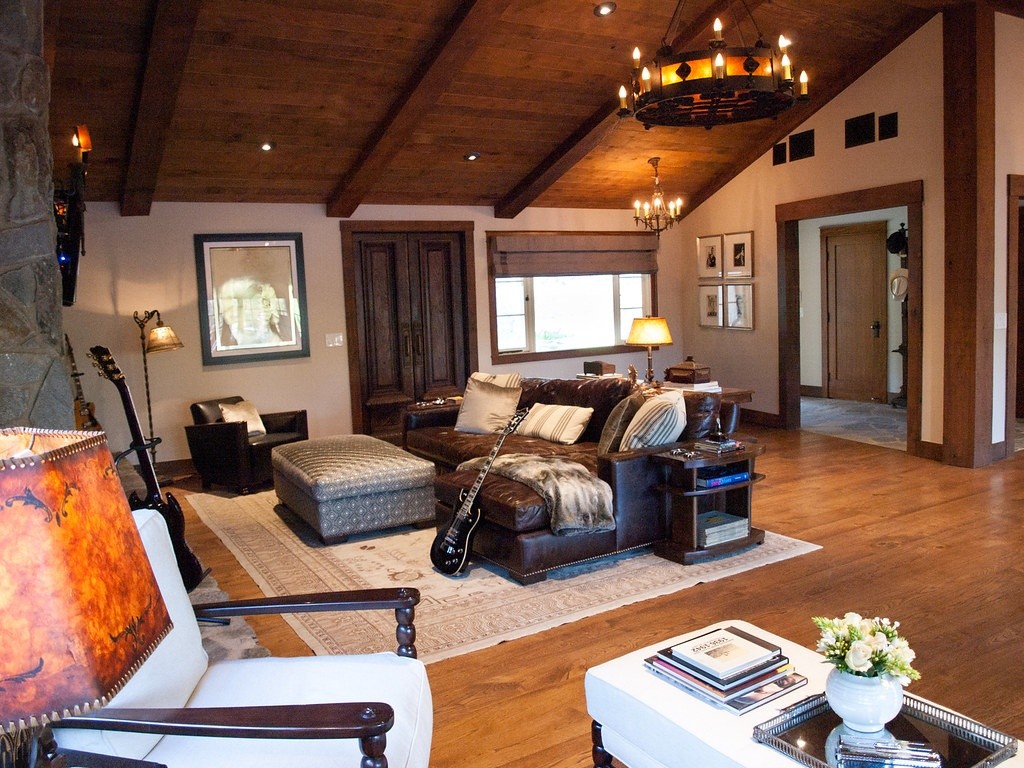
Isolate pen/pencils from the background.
[837,734,941,768]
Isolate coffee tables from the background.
[584,619,1024,768]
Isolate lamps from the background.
[0,426,175,768]
[632,156,683,240]
[625,315,674,393]
[133,308,183,488]
[615,0,812,129]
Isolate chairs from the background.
[49,505,433,768]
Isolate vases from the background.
[824,665,903,732]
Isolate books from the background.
[694,441,745,454]
[661,381,722,393]
[643,625,808,716]
[697,467,749,488]
[575,373,624,379]
[696,511,748,548]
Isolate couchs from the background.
[184,396,308,494]
[401,378,738,586]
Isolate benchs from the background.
[271,433,437,546]
[112,452,147,503]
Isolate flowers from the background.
[811,610,923,685]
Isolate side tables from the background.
[651,440,767,564]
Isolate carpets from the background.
[184,482,823,664]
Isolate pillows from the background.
[512,402,594,445]
[454,372,523,435]
[597,390,645,456]
[219,399,266,437]
[619,390,688,453]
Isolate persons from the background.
[707,247,716,267]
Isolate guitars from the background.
[64,333,103,432]
[86,344,204,595]
[627,365,638,382]
[429,407,530,577]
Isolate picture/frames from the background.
[695,231,753,279]
[697,282,755,331]
[191,231,311,366]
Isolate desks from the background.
[57,747,168,768]
[656,388,755,436]
